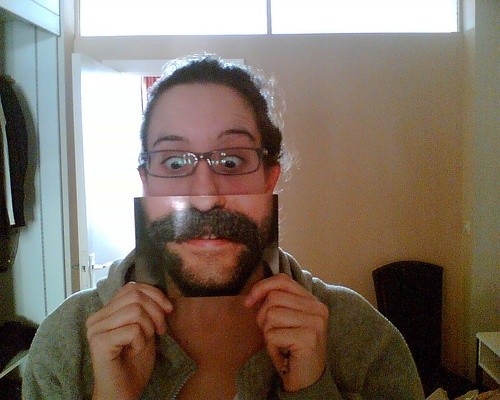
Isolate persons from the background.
[140,195,273,295]
[22,50,426,400]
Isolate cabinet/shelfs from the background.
[476,332,500,394]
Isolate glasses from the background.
[137,146,267,178]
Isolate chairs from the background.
[373,261,477,399]
